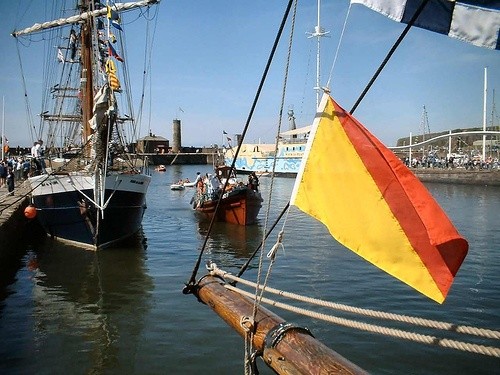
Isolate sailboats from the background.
[10,0,162,252]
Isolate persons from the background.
[0,140,46,196]
[400,152,500,170]
[70,29,81,63]
[178,175,258,202]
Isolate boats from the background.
[184,182,195,187]
[155,165,166,171]
[190,164,263,226]
[170,184,185,190]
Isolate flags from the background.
[289,91,470,304]
[106,5,124,93]
